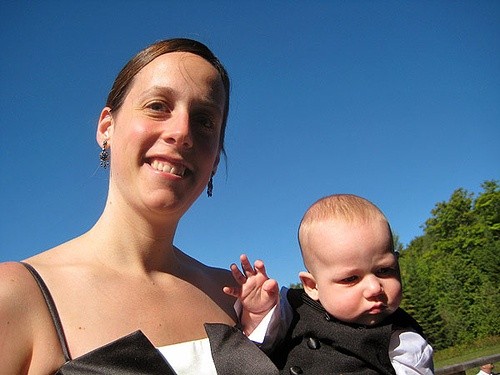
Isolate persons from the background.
[476,362,496,375]
[1,38,283,375]
[223,194,435,375]
